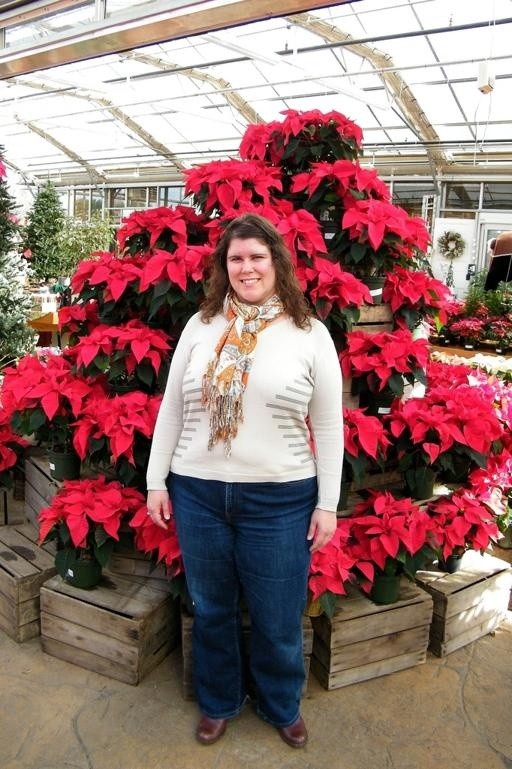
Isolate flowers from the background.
[130,245,215,328]
[67,249,141,320]
[467,366,512,431]
[486,320,512,348]
[114,206,205,250]
[207,203,323,275]
[425,360,476,393]
[62,319,176,395]
[242,110,365,173]
[74,393,165,478]
[341,330,431,398]
[437,231,465,258]
[425,483,505,561]
[338,486,425,596]
[326,198,427,276]
[376,262,449,328]
[425,386,504,472]
[180,159,285,217]
[449,317,485,344]
[289,164,390,217]
[307,518,359,620]
[58,301,99,344]
[128,500,181,582]
[305,406,394,482]
[286,255,372,321]
[0,354,84,451]
[381,396,478,472]
[36,471,144,554]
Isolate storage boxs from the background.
[40,571,176,684]
[0,523,69,643]
[412,549,511,656]
[24,455,87,536]
[314,574,433,692]
[181,608,314,697]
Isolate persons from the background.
[40,275,58,312]
[146,215,343,748]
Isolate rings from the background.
[147,512,152,516]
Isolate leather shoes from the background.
[279,715,307,748]
[196,717,226,743]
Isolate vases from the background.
[440,338,451,347]
[464,343,476,350]
[54,546,102,589]
[496,347,506,355]
[362,276,386,305]
[372,571,402,603]
[49,447,80,480]
[405,470,436,499]
[437,553,462,573]
[110,381,136,399]
[338,482,350,510]
[442,472,467,482]
